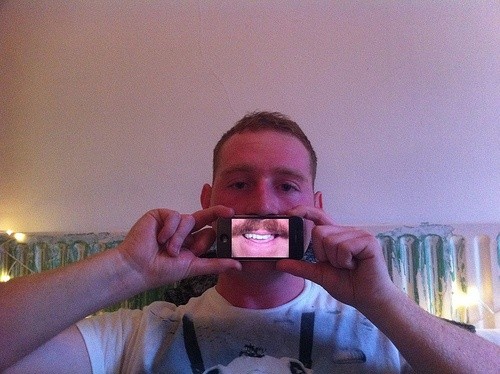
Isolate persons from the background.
[1,106,500,374]
[230,218,292,259]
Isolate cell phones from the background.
[215,214,304,261]
